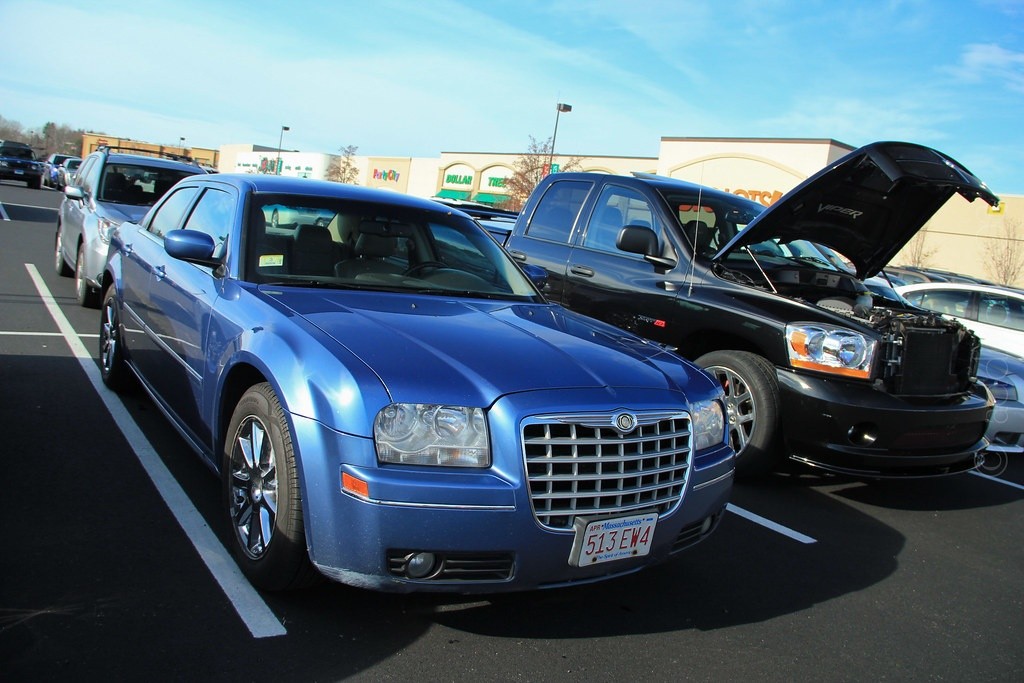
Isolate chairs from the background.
[291,224,341,276]
[629,219,652,228]
[153,173,180,202]
[223,207,281,275]
[596,206,623,252]
[989,304,1015,328]
[103,172,136,204]
[685,219,711,256]
[334,231,405,280]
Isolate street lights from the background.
[542,101,573,176]
[272,125,293,175]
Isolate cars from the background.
[870,265,1024,472]
[96,174,739,605]
[0,139,84,192]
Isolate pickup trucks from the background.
[439,140,1002,486]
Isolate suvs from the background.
[55,146,213,308]
